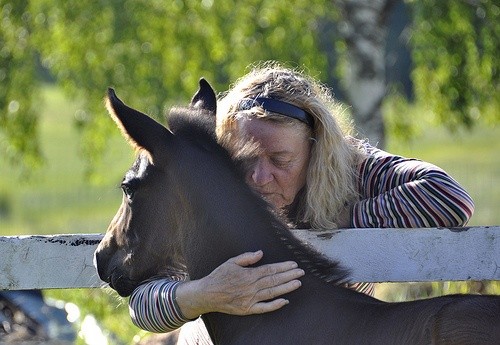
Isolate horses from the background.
[87,76,500,345]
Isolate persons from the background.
[129,59,476,345]
